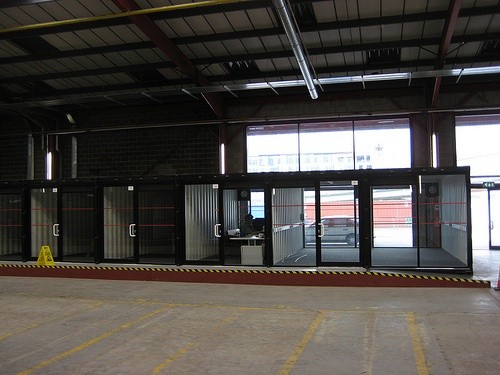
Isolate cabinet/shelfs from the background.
[229,235,266,266]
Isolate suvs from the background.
[305,215,376,245]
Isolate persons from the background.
[241,214,260,236]
